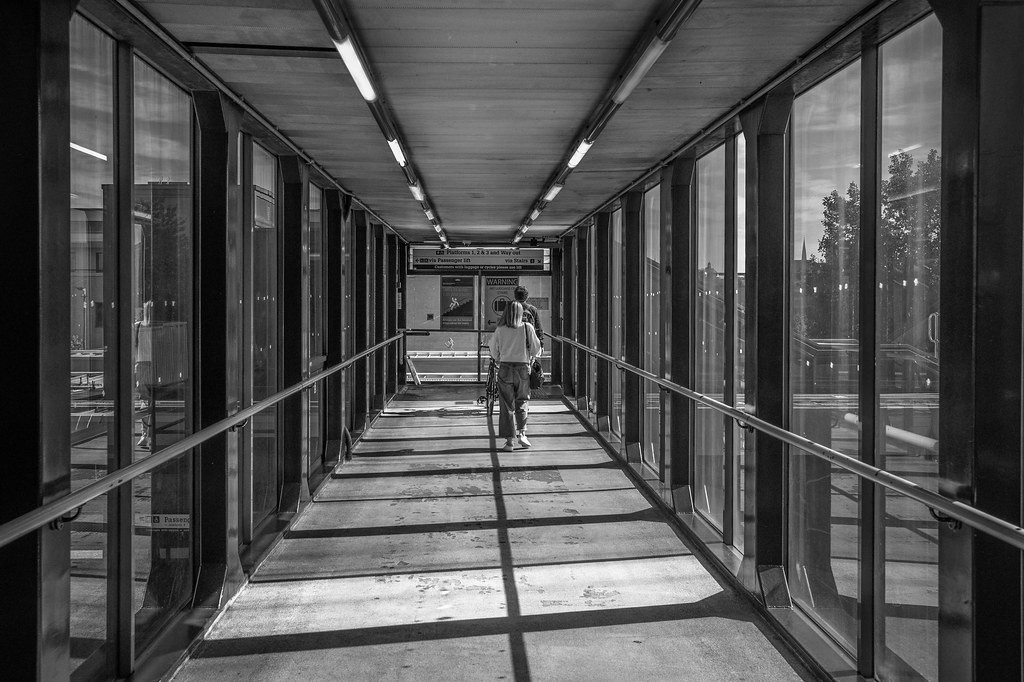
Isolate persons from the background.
[488,300,542,452]
[514,285,545,354]
[133,299,153,446]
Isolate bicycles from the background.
[477,342,499,417]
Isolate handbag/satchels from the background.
[530,360,545,390]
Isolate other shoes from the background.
[502,443,513,451]
[517,435,531,448]
